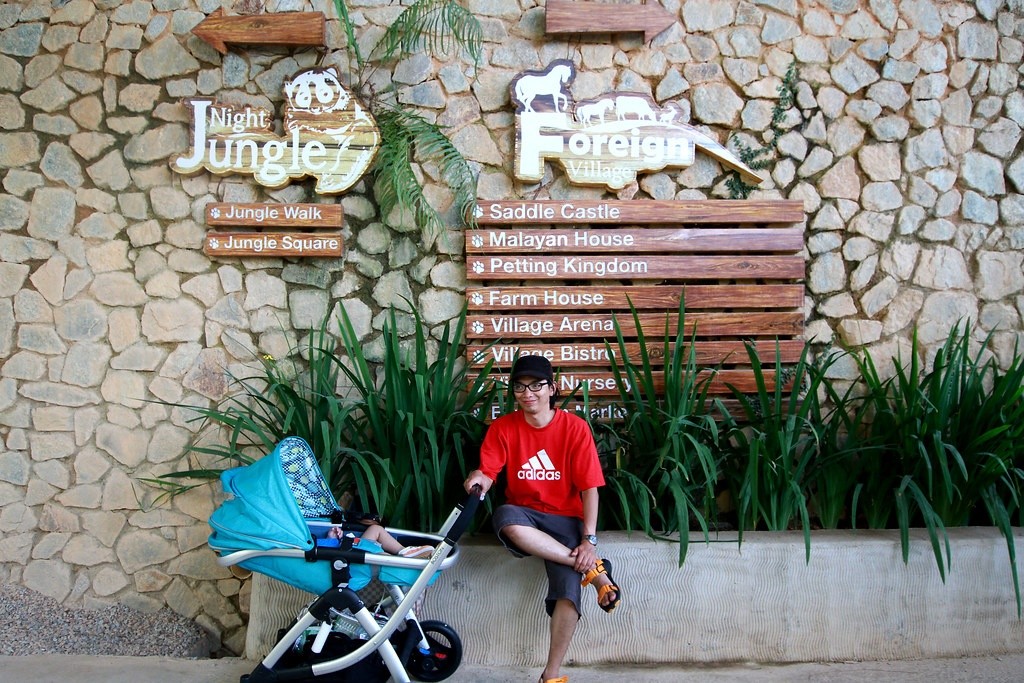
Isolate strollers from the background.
[207,437,482,683]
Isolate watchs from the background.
[582,535,597,545]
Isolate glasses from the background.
[511,382,549,394]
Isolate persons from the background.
[463,356,621,683]
[314,525,434,559]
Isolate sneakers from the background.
[398,545,435,559]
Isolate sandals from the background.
[580,557,622,614]
[538,674,569,683]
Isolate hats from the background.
[508,355,554,385]
